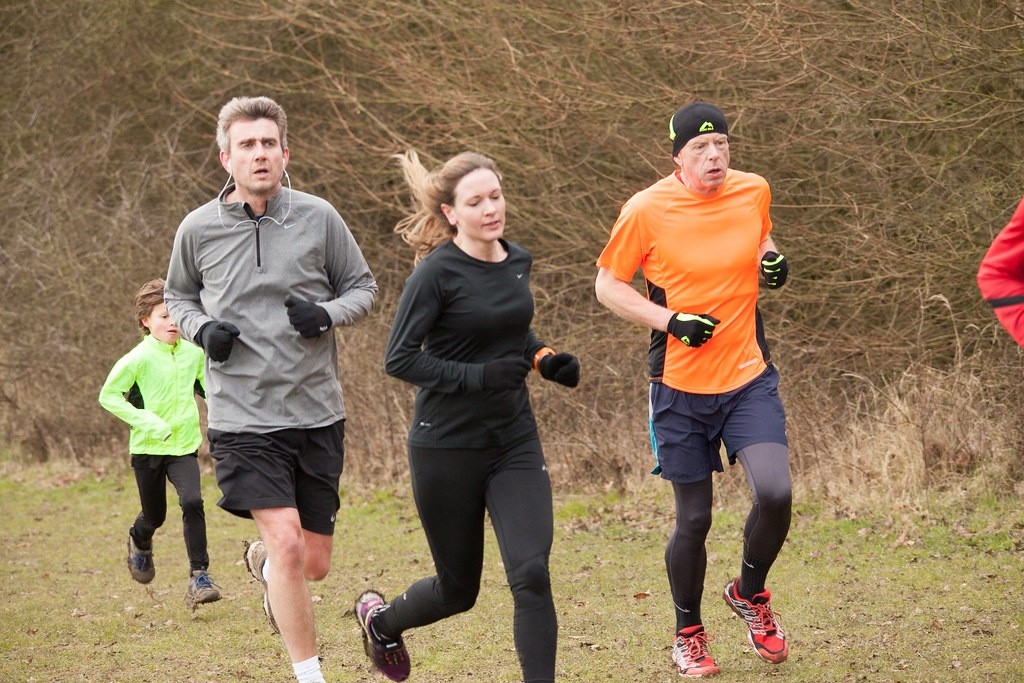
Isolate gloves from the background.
[484,360,533,391]
[195,322,240,364]
[283,295,331,339]
[668,313,721,349]
[540,352,580,388]
[761,250,787,291]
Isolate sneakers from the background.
[723,579,789,664]
[246,542,280,635]
[672,625,720,680]
[355,589,410,683]
[128,531,156,585]
[187,572,220,603]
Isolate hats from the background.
[668,104,728,157]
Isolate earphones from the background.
[283,157,286,171]
[227,160,231,170]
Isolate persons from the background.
[355,147,582,683]
[976,193,1024,355]
[164,96,377,683]
[99,278,228,604]
[594,102,792,678]
